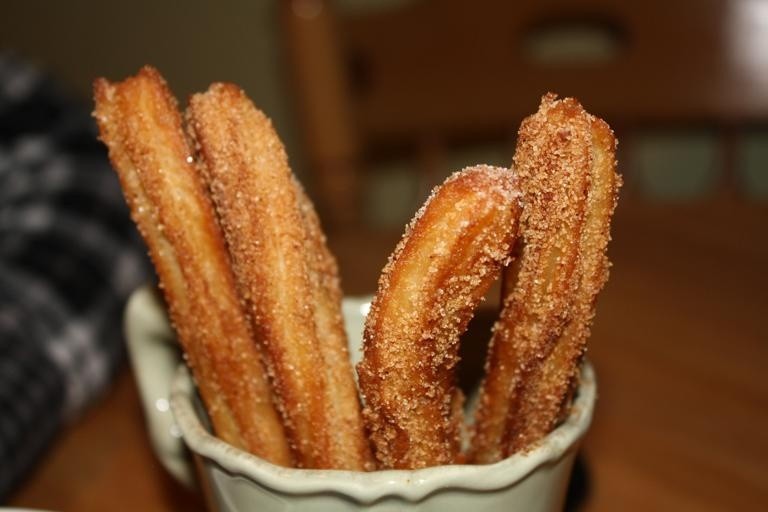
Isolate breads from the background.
[91,64,300,470]
[355,163,526,468]
[182,81,381,471]
[464,91,622,465]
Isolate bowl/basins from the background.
[122,281,598,512]
